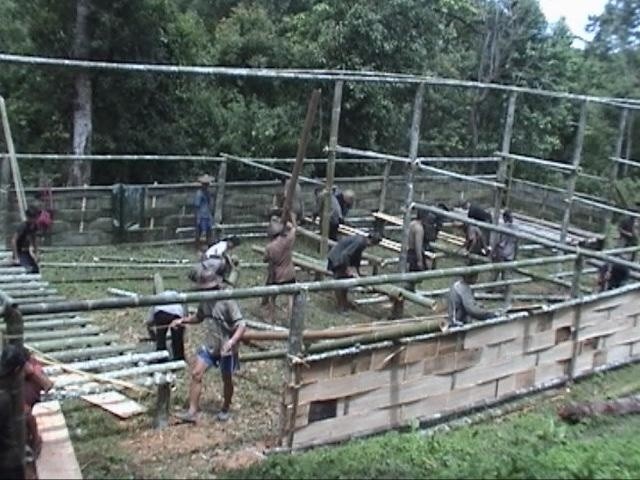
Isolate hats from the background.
[266,223,284,234]
[198,174,215,183]
[224,252,241,272]
[343,188,355,202]
[197,270,223,289]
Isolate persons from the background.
[0,344,31,479]
[145,289,188,375]
[262,175,519,327]
[596,246,629,292]
[11,203,42,275]
[193,174,214,250]
[200,236,240,262]
[35,208,54,232]
[616,206,640,247]
[188,253,242,283]
[168,268,246,422]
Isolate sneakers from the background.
[218,409,229,420]
[175,413,196,422]
